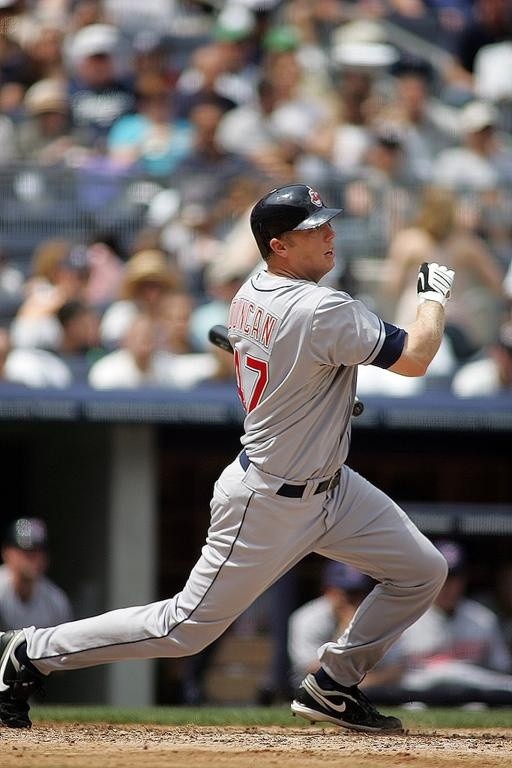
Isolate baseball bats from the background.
[208,325,364,416]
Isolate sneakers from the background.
[290,672,402,734]
[0,628,33,729]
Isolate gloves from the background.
[416,261,456,309]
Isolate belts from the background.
[239,450,341,498]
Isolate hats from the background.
[440,543,464,574]
[314,560,368,590]
[250,182,345,258]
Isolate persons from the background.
[0,0,512,715]
[1,181,457,735]
